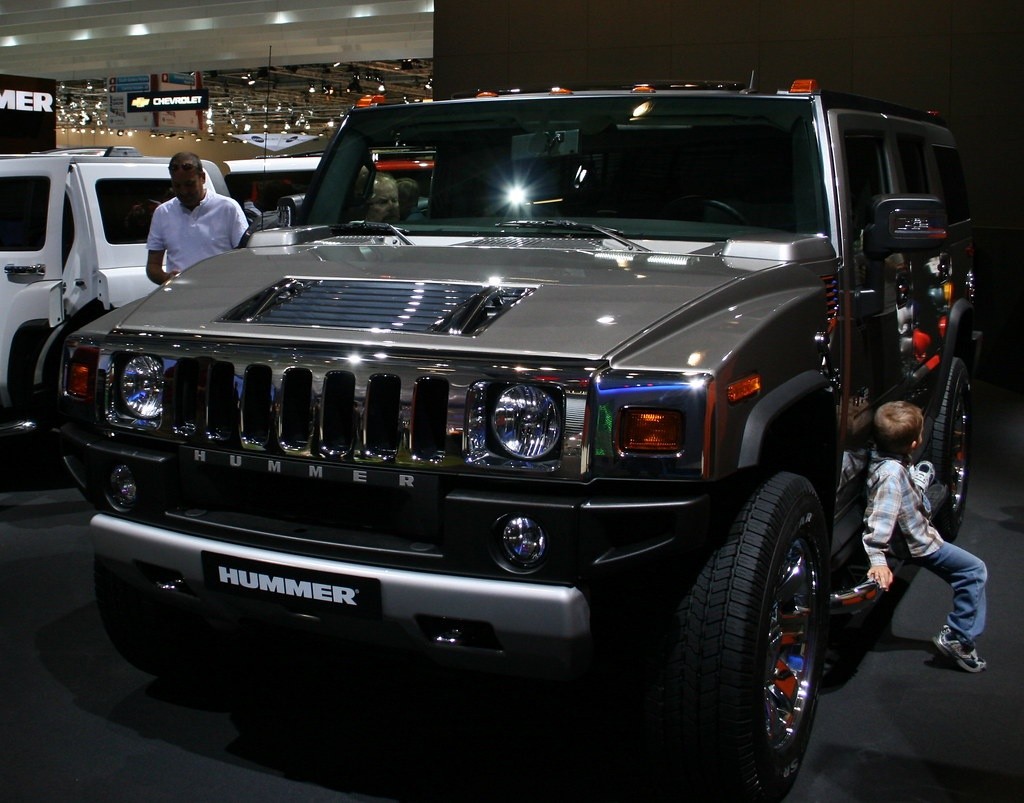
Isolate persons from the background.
[147,151,251,285]
[860,400,989,673]
[365,171,428,223]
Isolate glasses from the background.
[168,163,200,172]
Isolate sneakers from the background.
[912,461,935,492]
[932,625,986,673]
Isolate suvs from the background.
[0,144,321,464]
[49,76,984,802]
